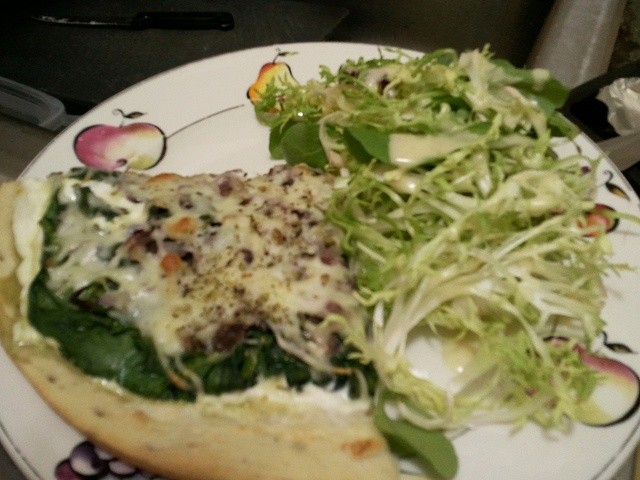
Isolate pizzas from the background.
[0,163,401,480]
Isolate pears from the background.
[526,336,639,426]
[246,48,300,103]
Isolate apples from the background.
[74,123,166,170]
[578,170,631,236]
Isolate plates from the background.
[1,40,640,479]
